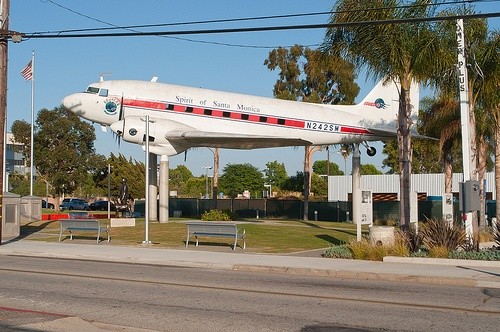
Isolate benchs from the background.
[185,223,246,252]
[58,218,111,244]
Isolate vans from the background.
[59,198,89,210]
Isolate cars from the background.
[42,199,55,209]
[89,200,116,211]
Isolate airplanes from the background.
[60,67,440,160]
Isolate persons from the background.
[119,178,128,218]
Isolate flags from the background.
[20,60,32,81]
